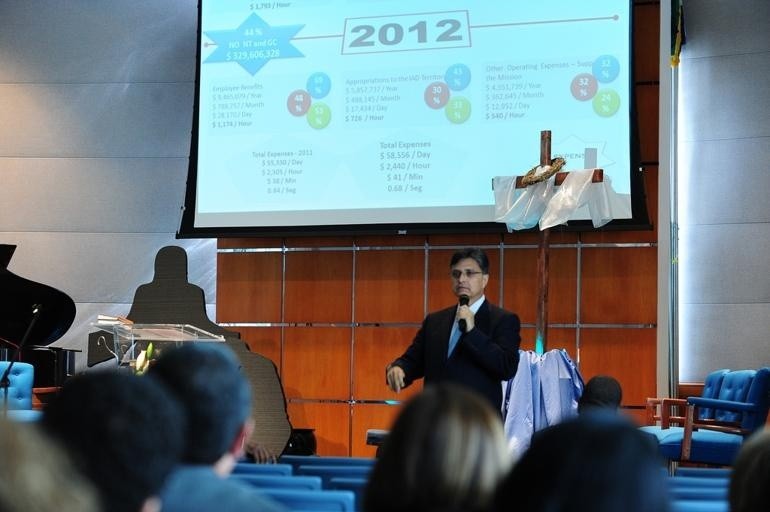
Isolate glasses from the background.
[448,268,484,278]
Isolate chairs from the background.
[1,361,770,512]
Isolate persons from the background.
[36,367,190,511]
[0,407,100,511]
[727,421,768,508]
[358,385,512,510]
[577,372,621,414]
[242,435,276,464]
[140,343,254,477]
[495,409,675,510]
[384,249,520,421]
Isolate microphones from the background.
[458,294,470,331]
